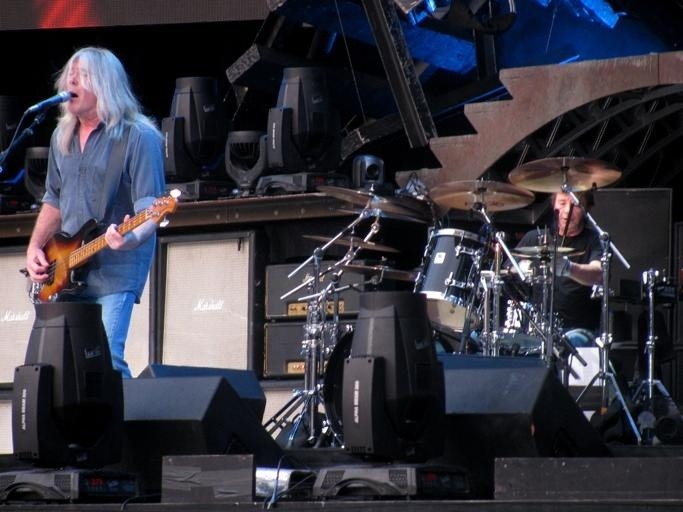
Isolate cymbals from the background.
[302,233,398,252]
[429,179,535,213]
[316,185,428,223]
[338,263,417,281]
[508,157,622,194]
[508,247,586,259]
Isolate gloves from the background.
[518,259,533,280]
[548,256,571,278]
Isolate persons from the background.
[23,46,167,378]
[469,191,614,347]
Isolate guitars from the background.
[32,196,178,304]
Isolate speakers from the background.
[123,375,254,453]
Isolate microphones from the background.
[28,89,72,112]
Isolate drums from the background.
[473,271,565,354]
[413,228,503,333]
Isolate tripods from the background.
[632,267,683,420]
[261,277,346,449]
[575,232,643,445]
